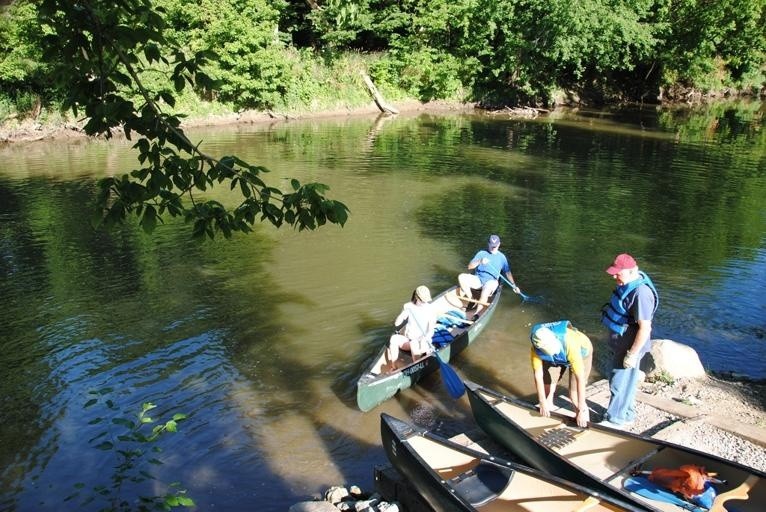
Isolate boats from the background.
[378,412,646,511]
[463,380,766,512]
[356,279,504,414]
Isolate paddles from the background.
[406,307,465,398]
[488,261,542,304]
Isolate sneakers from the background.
[465,300,479,322]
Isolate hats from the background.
[415,286,433,302]
[606,253,637,275]
[532,327,562,356]
[487,234,501,249]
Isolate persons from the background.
[530,321,593,428]
[458,235,521,323]
[594,253,660,424]
[386,286,437,374]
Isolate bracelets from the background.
[578,407,587,413]
[511,282,515,286]
